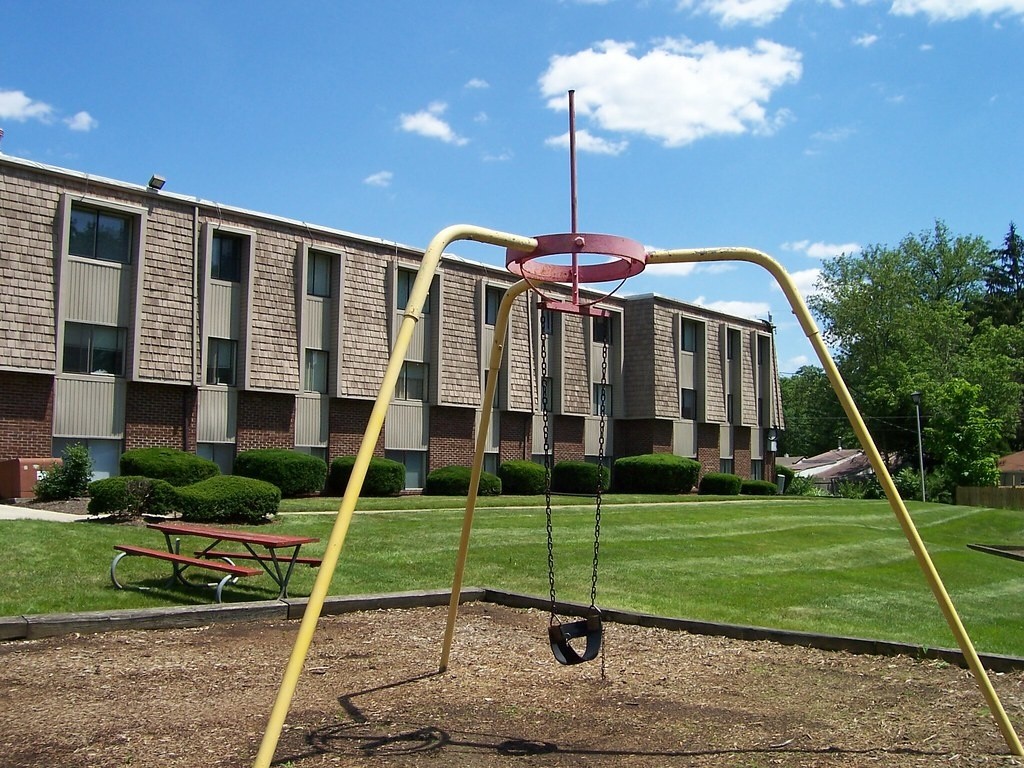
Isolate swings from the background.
[539,306,607,666]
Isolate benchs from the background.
[192,551,323,592]
[109,545,266,605]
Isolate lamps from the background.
[148,174,166,191]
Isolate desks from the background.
[145,522,321,599]
